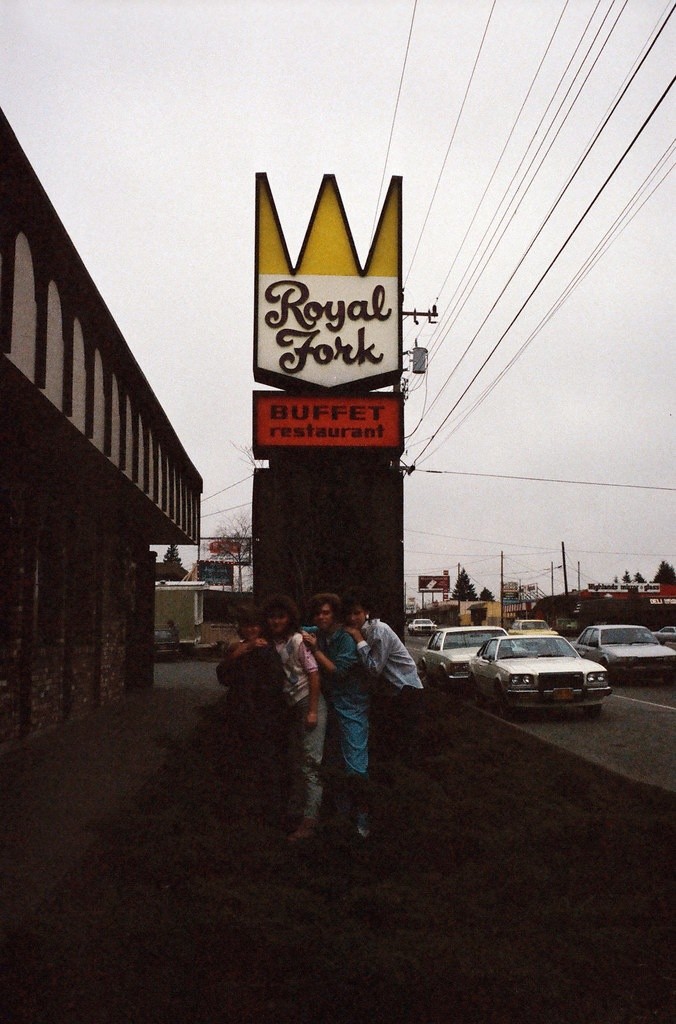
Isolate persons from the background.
[216,604,285,825]
[167,619,179,646]
[339,586,424,832]
[228,591,328,846]
[299,593,371,837]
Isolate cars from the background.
[407,618,438,636]
[569,625,676,686]
[510,619,560,643]
[651,626,676,642]
[416,626,528,692]
[468,635,614,720]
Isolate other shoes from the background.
[348,790,371,825]
[283,817,322,851]
